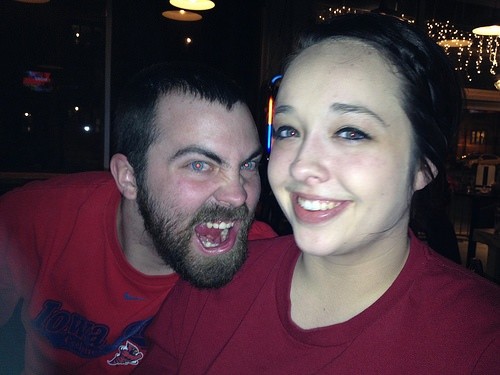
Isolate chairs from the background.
[469,218,500,283]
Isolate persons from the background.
[132,13,498,374]
[1,62,281,375]
